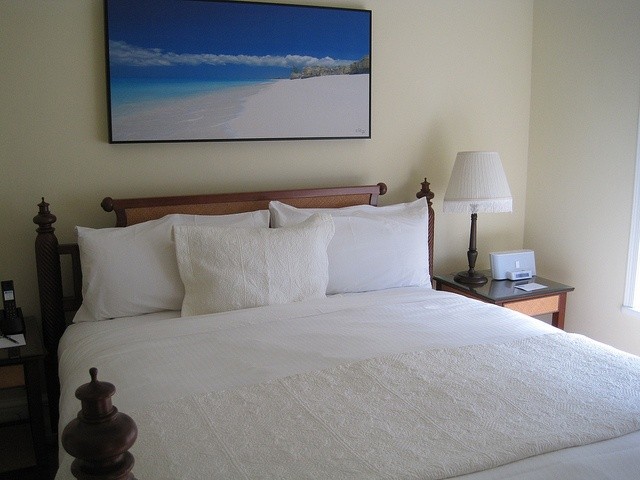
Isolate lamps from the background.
[443,151,513,286]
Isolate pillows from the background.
[269,197,432,296]
[173,211,337,319]
[71,209,270,323]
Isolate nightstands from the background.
[1,314,46,442]
[432,268,575,331]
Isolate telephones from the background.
[0,280,23,333]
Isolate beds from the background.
[32,183,639,479]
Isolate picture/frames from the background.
[105,0,373,144]
